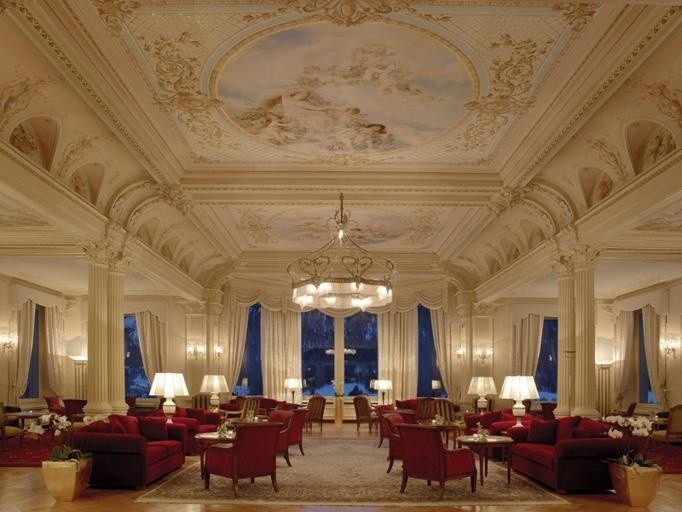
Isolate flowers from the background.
[27,413,93,462]
[599,414,661,468]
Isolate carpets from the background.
[133,432,572,505]
[645,439,682,474]
[1,431,69,467]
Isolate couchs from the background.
[373,404,393,449]
[220,397,286,419]
[464,409,555,461]
[0,401,25,449]
[62,399,87,423]
[204,422,284,499]
[65,415,187,490]
[43,394,64,416]
[508,416,655,495]
[147,407,228,456]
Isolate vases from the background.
[608,462,663,508]
[41,458,93,502]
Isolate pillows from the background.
[107,414,139,435]
[138,417,169,441]
[526,418,552,444]
[550,415,582,442]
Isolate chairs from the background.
[353,396,380,434]
[230,398,261,431]
[275,409,312,457]
[414,398,444,449]
[432,399,462,449]
[304,395,327,434]
[381,413,405,474]
[394,423,477,501]
[275,412,292,467]
[655,404,682,448]
[607,401,636,417]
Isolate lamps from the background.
[199,375,229,413]
[285,192,395,313]
[374,380,392,406]
[148,372,190,423]
[284,378,303,404]
[498,375,540,428]
[466,376,498,415]
